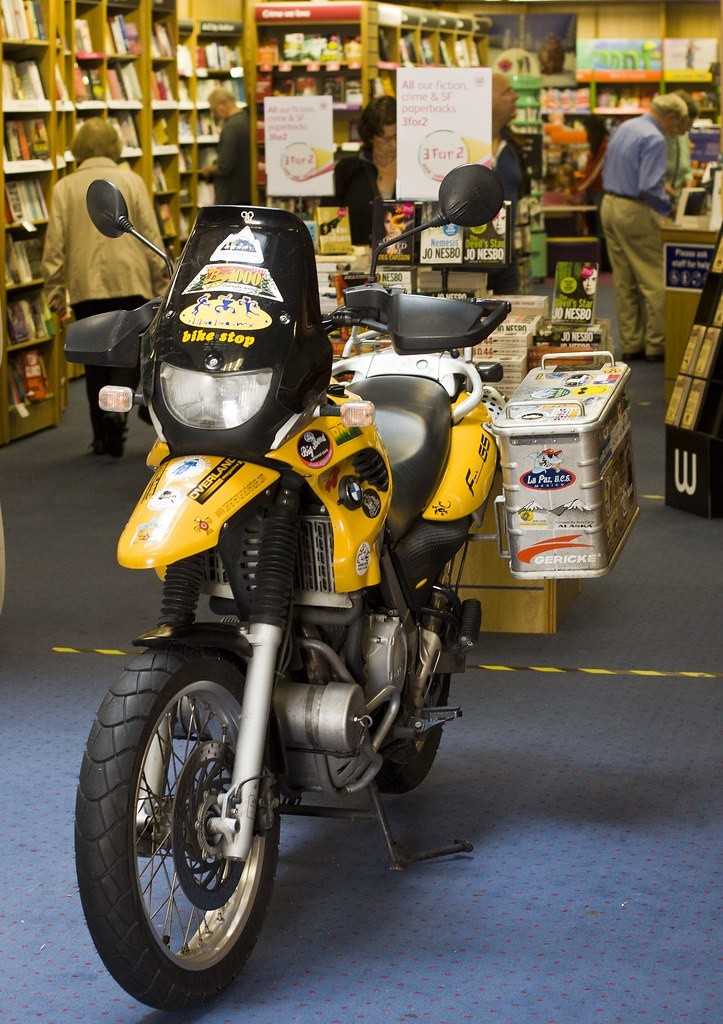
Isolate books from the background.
[0,0,653,406]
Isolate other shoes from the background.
[104,411,128,458]
[646,355,664,362]
[86,439,106,454]
[623,348,645,360]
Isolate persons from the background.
[41,116,167,456]
[488,72,531,293]
[600,90,699,363]
[203,88,252,206]
[318,95,397,246]
[577,116,612,272]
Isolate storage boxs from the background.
[490,351,641,581]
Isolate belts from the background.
[606,191,635,200]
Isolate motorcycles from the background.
[64,162,512,1015]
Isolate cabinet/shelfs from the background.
[0,0,723,635]
